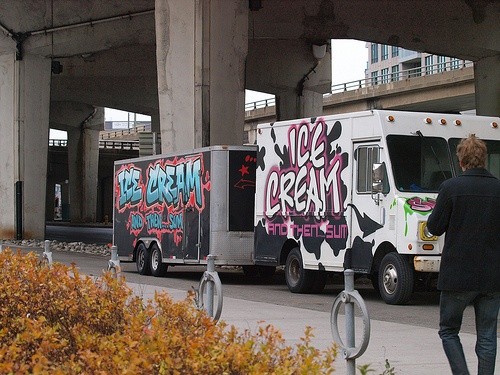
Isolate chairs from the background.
[430,170,457,191]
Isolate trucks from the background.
[251,109,500,306]
